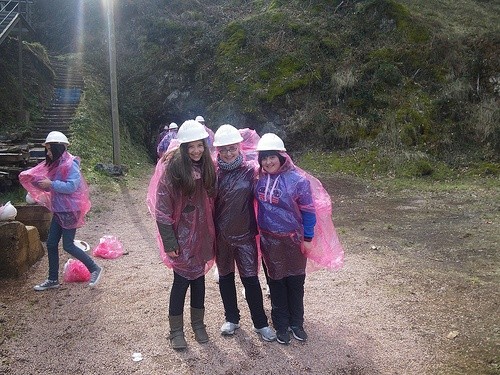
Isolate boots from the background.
[190,306,208,342]
[167,314,186,348]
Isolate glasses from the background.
[219,145,239,154]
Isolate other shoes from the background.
[266,283,271,295]
[243,286,247,299]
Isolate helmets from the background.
[0,201,17,221]
[255,133,286,151]
[177,120,209,143]
[169,123,178,129]
[41,131,70,147]
[26,192,37,204]
[195,116,205,121]
[164,126,168,129]
[212,124,243,146]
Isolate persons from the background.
[209,124,277,342]
[157,122,178,158]
[195,116,215,153]
[252,133,317,344]
[19,131,102,290]
[154,119,218,350]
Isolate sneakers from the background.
[220,320,240,334]
[89,267,103,287]
[253,325,277,341]
[288,325,308,340]
[275,328,291,346]
[33,279,60,290]
